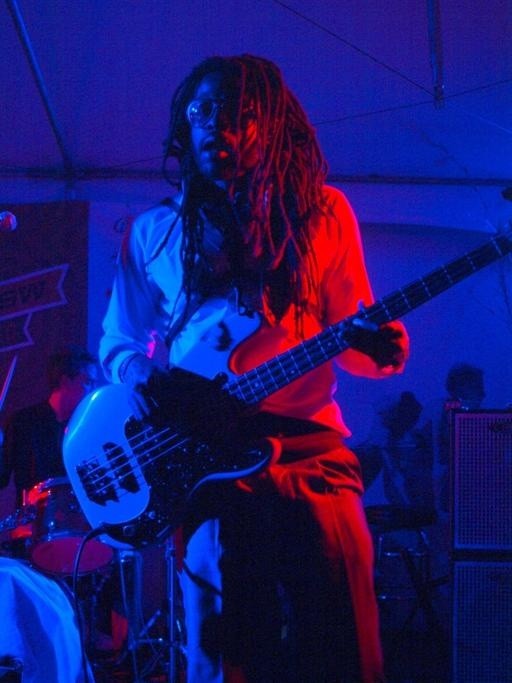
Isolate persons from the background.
[98,56,407,683]
[359,368,483,514]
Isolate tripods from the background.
[103,530,186,683]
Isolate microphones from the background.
[0,210,17,235]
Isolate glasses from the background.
[186,99,255,132]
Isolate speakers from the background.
[444,404,512,683]
